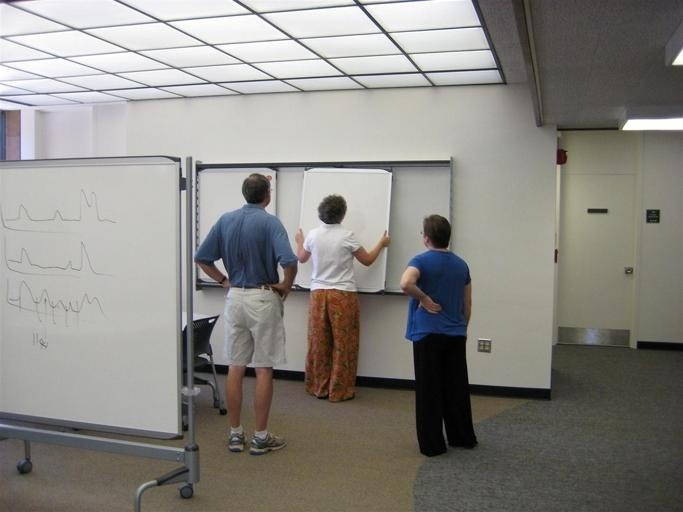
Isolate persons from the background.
[294,193,391,403]
[193,172,302,455]
[398,213,478,457]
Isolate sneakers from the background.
[229,433,249,453]
[249,433,289,455]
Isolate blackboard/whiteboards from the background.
[294,166,393,293]
[0,154,185,440]
[194,167,276,284]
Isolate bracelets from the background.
[218,276,227,285]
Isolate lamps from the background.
[664,24,683,69]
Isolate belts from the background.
[232,284,273,292]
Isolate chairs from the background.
[180,314,227,430]
